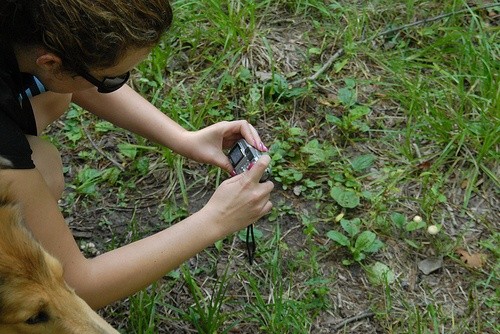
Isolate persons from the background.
[0,0,277,314]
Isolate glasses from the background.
[59,50,130,94]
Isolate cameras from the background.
[226,138,272,183]
